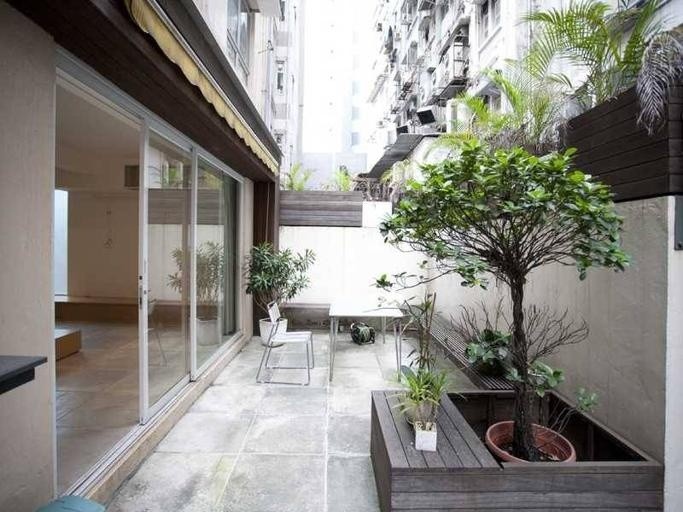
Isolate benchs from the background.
[396,303,534,390]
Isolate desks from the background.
[0,355,47,394]
[327,295,404,383]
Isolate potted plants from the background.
[385,362,469,452]
[368,134,636,466]
[239,240,317,349]
[364,292,442,425]
[164,240,226,345]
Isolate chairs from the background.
[147,298,167,368]
[253,299,315,387]
